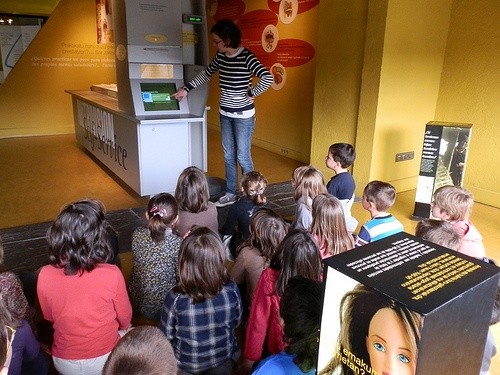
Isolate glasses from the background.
[214,39,223,44]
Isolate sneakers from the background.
[214,193,236,207]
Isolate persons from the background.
[128,143,404,375]
[0,248,44,375]
[448,127,473,188]
[37,199,134,375]
[171,18,274,207]
[416,184,500,375]
[102,325,178,375]
[319,283,425,375]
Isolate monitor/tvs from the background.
[138,81,183,112]
